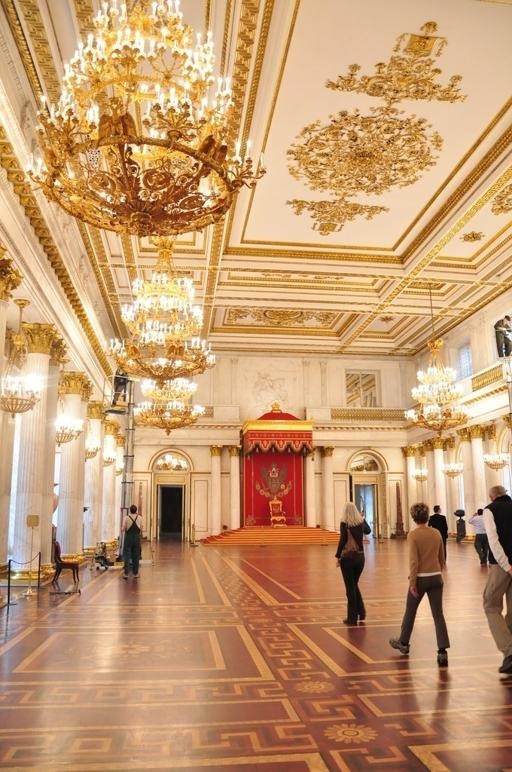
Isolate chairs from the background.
[269,496,286,527]
[52,541,80,583]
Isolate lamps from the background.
[26,0,267,236]
[0,300,43,418]
[104,457,114,466]
[415,442,428,482]
[441,433,463,479]
[56,359,82,447]
[404,283,468,431]
[485,420,506,471]
[134,379,204,436]
[103,237,215,381]
[85,447,98,462]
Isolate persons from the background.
[481,485,512,674]
[335,503,371,625]
[388,502,451,666]
[468,509,488,564]
[494,315,512,357]
[120,504,143,579]
[428,505,448,565]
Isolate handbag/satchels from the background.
[340,526,360,558]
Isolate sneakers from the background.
[389,639,411,654]
[437,650,448,667]
[498,654,512,674]
[122,573,140,579]
[342,618,357,624]
